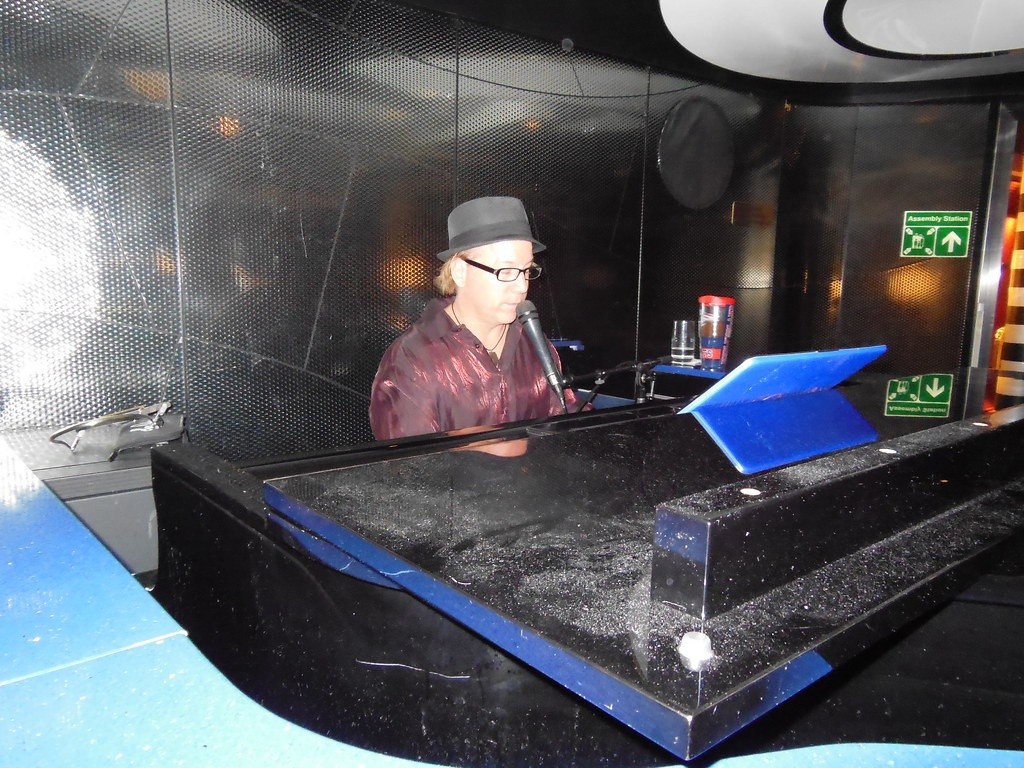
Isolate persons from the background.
[368,196,593,443]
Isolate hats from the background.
[436,197,546,263]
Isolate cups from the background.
[670,319,696,362]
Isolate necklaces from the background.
[451,301,505,367]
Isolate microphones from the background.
[515,300,564,401]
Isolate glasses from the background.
[459,255,542,282]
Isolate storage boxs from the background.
[3,411,191,576]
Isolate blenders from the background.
[697,295,736,372]
